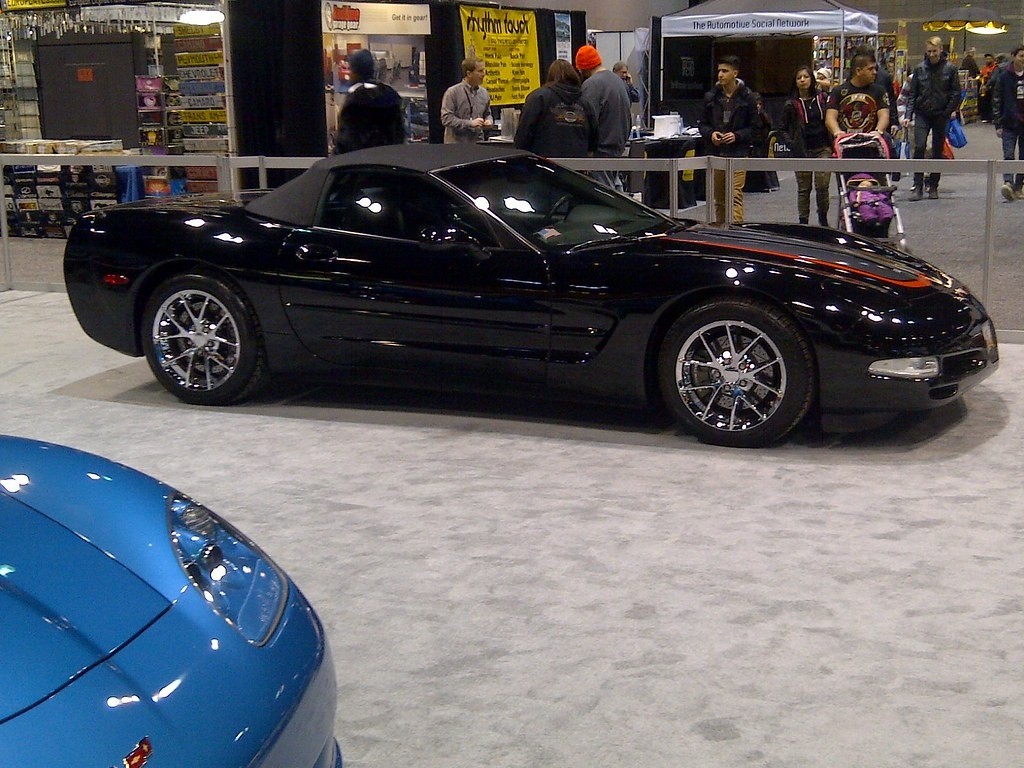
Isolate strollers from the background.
[834,132,909,255]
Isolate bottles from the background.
[635,115,641,127]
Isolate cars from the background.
[64,144,1000,448]
[1,435,343,768]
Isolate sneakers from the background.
[908,187,923,200]
[929,187,937,199]
[1000,183,1016,201]
[1016,191,1024,199]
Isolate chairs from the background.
[743,131,782,193]
[446,173,486,237]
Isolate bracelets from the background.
[834,131,844,136]
[876,129,883,134]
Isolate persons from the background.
[699,55,758,223]
[334,48,405,156]
[440,56,493,143]
[904,36,961,201]
[825,52,890,138]
[897,73,933,193]
[612,62,640,103]
[755,96,771,140]
[850,181,893,225]
[856,45,900,181]
[960,47,1007,124]
[992,44,1024,200]
[776,66,833,227]
[575,45,631,189]
[516,59,599,157]
[815,68,834,92]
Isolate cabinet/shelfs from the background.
[810,34,896,83]
[134,73,182,154]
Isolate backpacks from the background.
[541,83,589,158]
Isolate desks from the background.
[630,129,702,209]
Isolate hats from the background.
[350,50,375,80]
[576,46,601,70]
[817,68,832,78]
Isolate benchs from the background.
[331,175,438,238]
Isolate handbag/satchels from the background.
[945,118,967,149]
[895,125,910,159]
[942,138,955,160]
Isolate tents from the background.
[644,0,879,128]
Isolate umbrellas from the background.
[923,3,1008,51]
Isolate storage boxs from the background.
[0,138,122,155]
